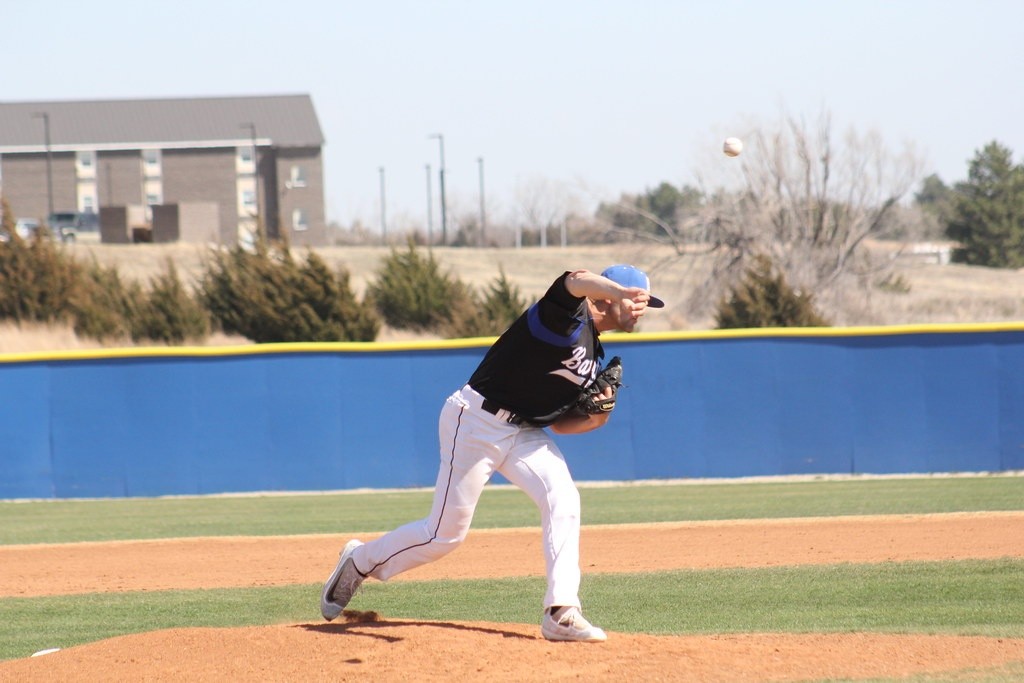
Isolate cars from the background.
[0,218,40,242]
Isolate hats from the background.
[601,264,664,308]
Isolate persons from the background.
[321,266,664,643]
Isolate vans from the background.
[41,211,102,244]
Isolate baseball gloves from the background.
[576,356,628,413]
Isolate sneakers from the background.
[541,606,607,643]
[321,539,364,622]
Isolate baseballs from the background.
[723,137,744,158]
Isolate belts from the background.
[482,399,525,426]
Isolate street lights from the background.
[29,111,54,244]
[474,156,487,248]
[239,120,262,251]
[377,165,386,247]
[427,132,448,247]
[424,163,434,248]
